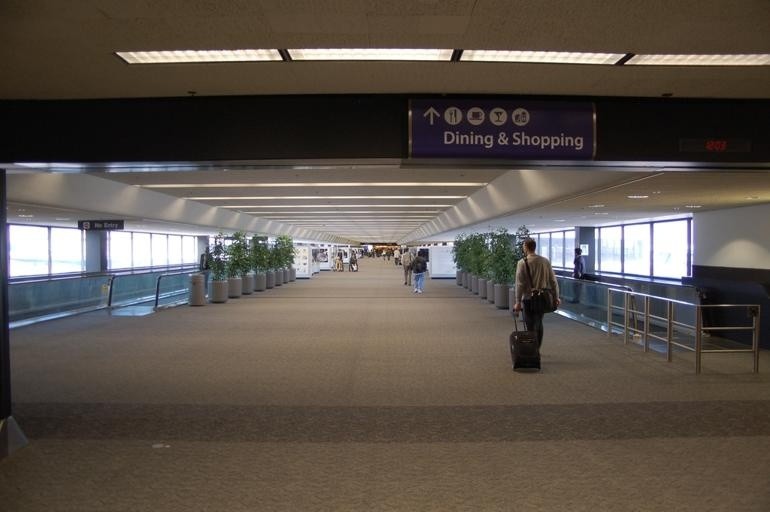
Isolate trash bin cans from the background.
[189,274,207,306]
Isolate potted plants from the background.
[209,234,296,304]
[454,229,528,315]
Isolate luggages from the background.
[510,308,542,370]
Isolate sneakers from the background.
[414,288,425,294]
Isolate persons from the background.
[512,237,561,348]
[570,246,586,303]
[334,247,401,272]
[410,251,427,294]
[401,248,415,285]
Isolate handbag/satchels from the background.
[417,263,426,273]
[409,261,413,270]
[532,286,558,313]
